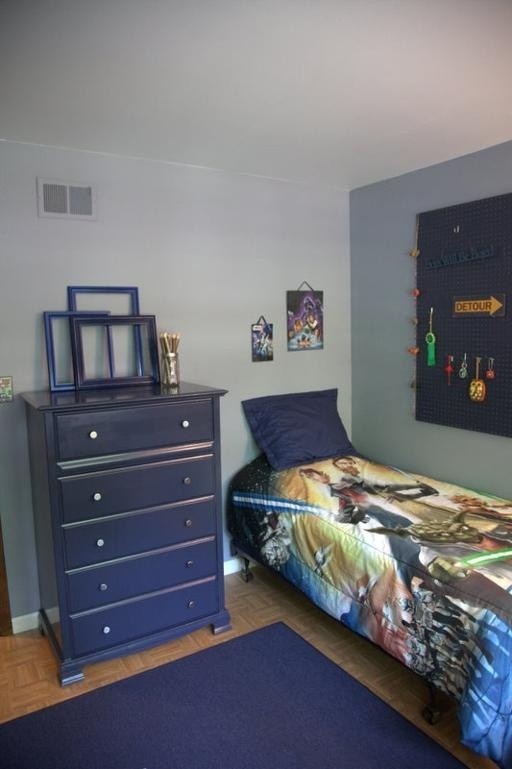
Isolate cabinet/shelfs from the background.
[20,379,228,687]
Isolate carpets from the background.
[0,619,469,769]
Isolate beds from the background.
[225,452,511,768]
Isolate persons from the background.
[297,455,512,628]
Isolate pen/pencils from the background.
[159,333,180,386]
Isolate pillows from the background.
[242,387,361,472]
